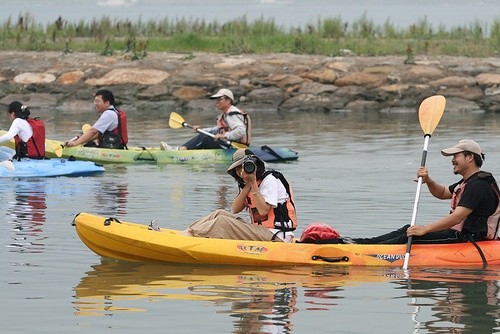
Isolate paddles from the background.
[403,95,446,269]
[54,124,92,157]
[0,154,16,171]
[168,111,248,149]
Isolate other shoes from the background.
[150,219,161,233]
[341,236,355,244]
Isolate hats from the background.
[210,89,234,101]
[441,139,485,162]
[225,148,265,178]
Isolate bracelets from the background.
[252,191,260,194]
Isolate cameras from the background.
[244,155,257,173]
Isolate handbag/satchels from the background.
[101,134,123,150]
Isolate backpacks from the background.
[295,220,342,242]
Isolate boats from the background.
[0,144,106,179]
[72,210,500,277]
[0,130,301,166]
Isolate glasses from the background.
[214,96,225,101]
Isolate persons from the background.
[180,88,251,150]
[342,140,500,244]
[177,149,297,242]
[66,89,128,149]
[1,101,45,161]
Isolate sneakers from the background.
[159,140,174,151]
